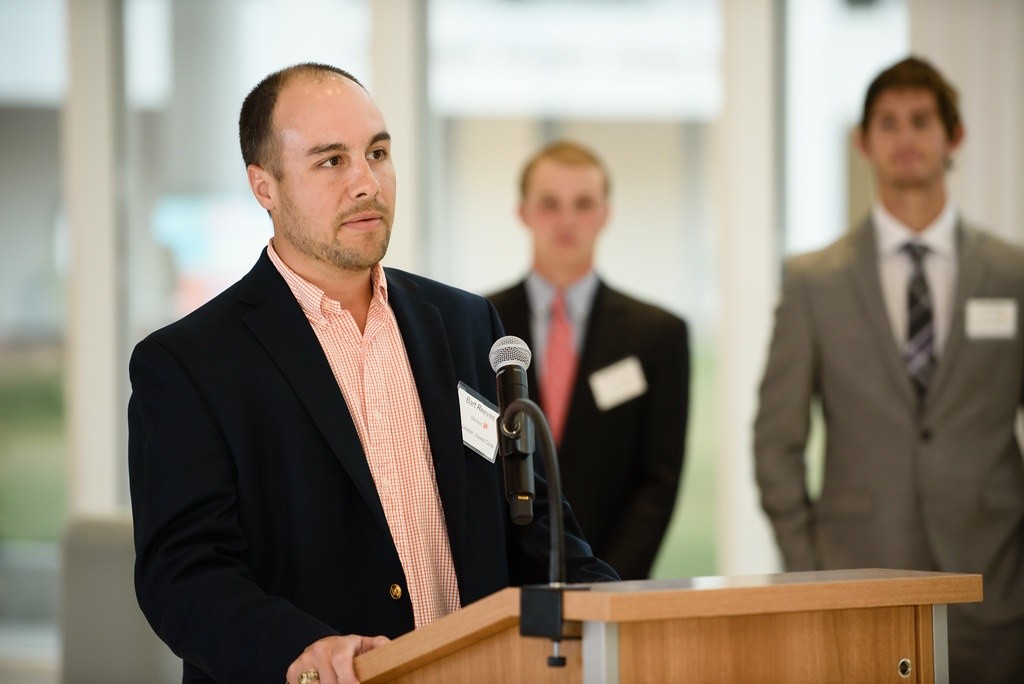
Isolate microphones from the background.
[488,336,537,525]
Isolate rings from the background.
[298,670,319,684]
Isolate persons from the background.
[754,57,1024,684]
[127,62,622,684]
[483,142,690,581]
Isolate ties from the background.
[902,243,939,398]
[542,293,579,442]
